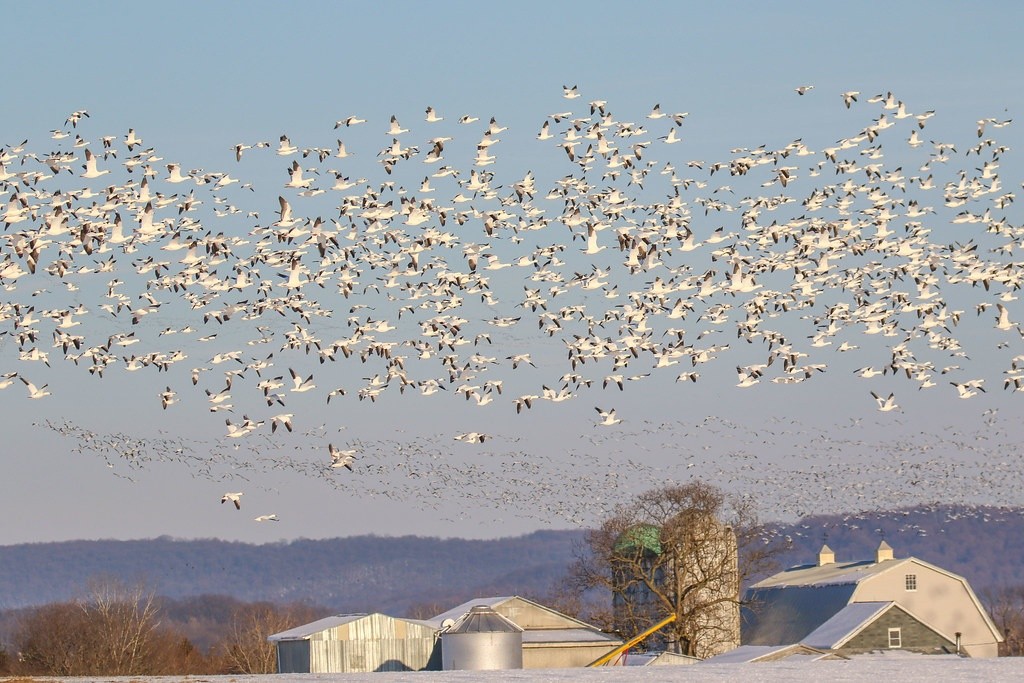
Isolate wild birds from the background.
[0,83,1022,540]
